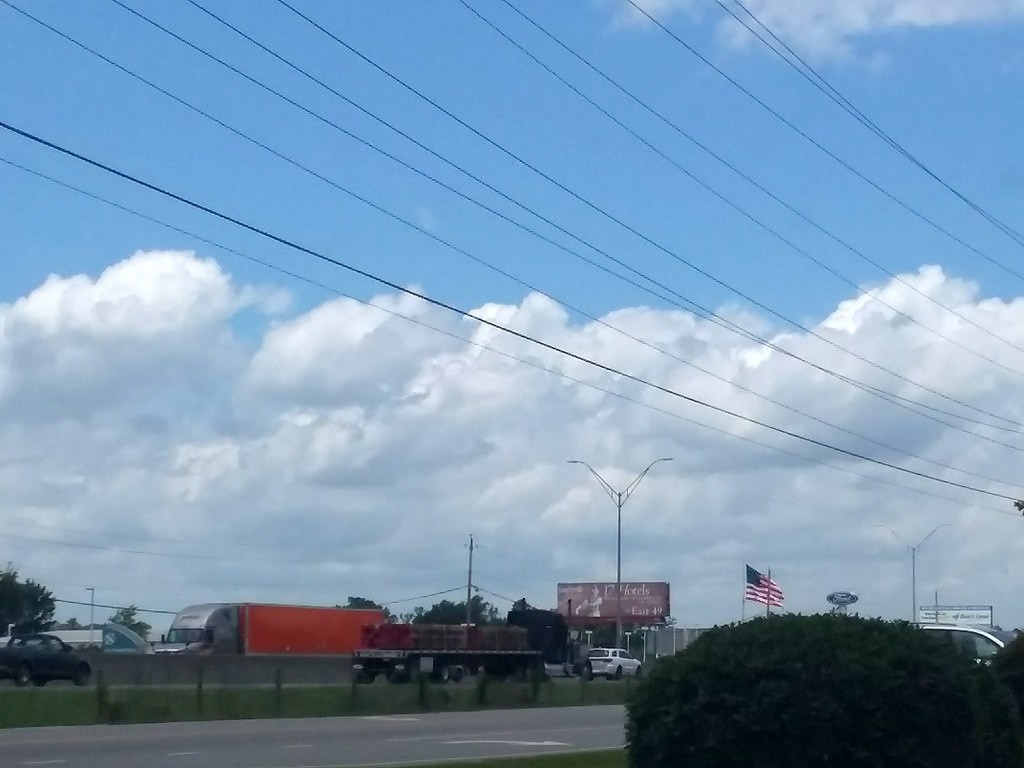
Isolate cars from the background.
[589,647,642,681]
[0,632,92,688]
[917,622,1017,666]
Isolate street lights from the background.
[85,587,95,630]
[566,458,675,648]
[877,523,953,622]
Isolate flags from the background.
[745,564,784,607]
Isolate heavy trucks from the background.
[353,607,571,685]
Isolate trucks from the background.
[153,602,385,657]
[0,619,157,655]
